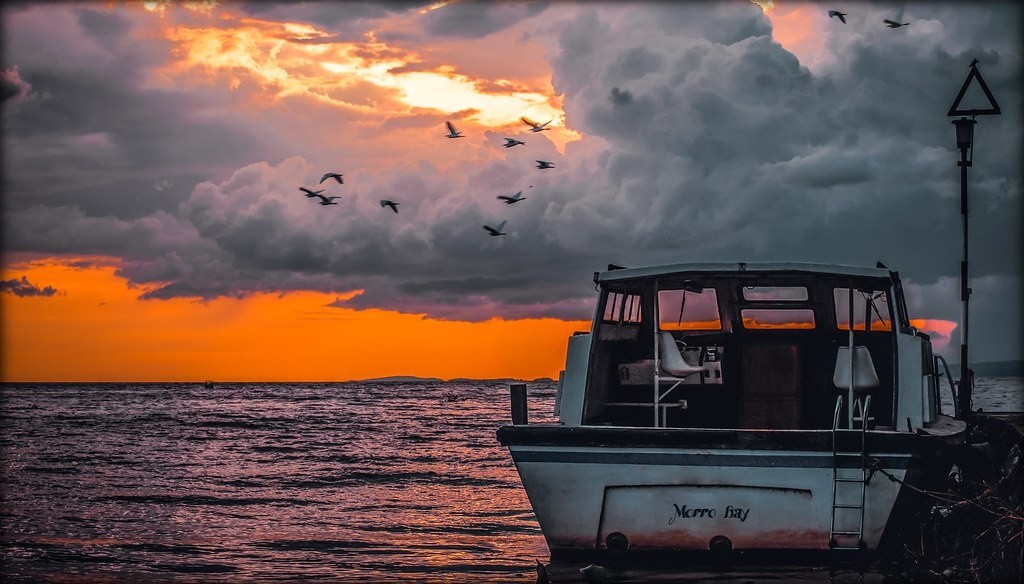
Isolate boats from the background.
[495,261,967,559]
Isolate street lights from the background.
[952,117,977,414]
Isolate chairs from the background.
[833,344,881,426]
[737,343,802,429]
[654,330,707,427]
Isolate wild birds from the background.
[496,191,527,205]
[444,120,466,138]
[502,137,526,147]
[298,186,341,205]
[380,199,400,214]
[828,9,847,25]
[534,160,557,169]
[319,172,344,185]
[522,118,552,132]
[884,18,910,29]
[482,220,507,236]
